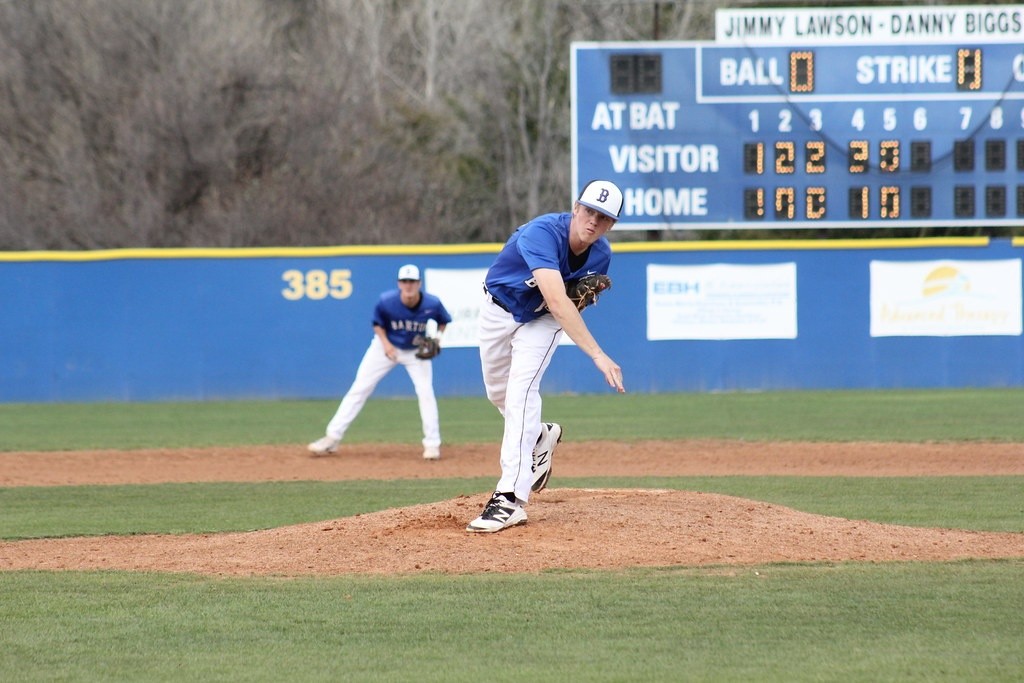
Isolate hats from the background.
[397,264,421,281]
[577,180,623,221]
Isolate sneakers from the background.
[423,448,440,459]
[531,422,563,493]
[307,436,338,455]
[465,491,527,533]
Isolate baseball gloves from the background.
[542,274,612,314]
[415,336,441,360]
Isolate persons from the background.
[466,179,625,533]
[307,264,451,460]
